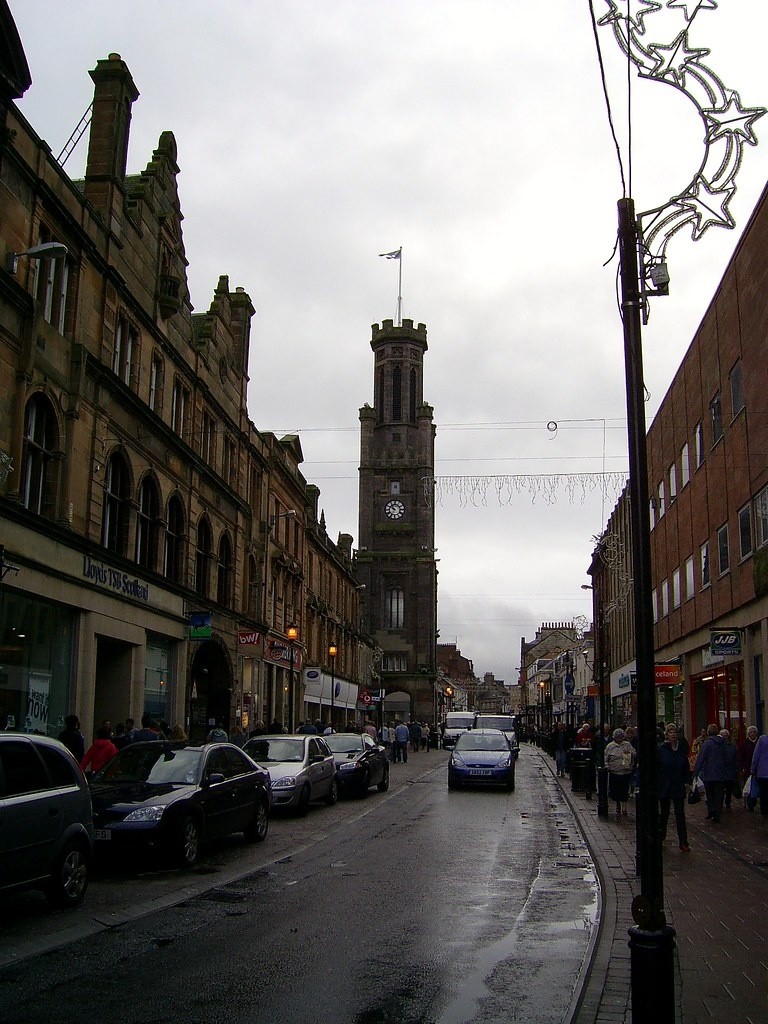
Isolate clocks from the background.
[384,499,406,520]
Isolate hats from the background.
[141,712,153,725]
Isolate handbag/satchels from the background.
[742,775,760,798]
[622,752,632,770]
[688,776,707,804]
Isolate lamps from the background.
[270,509,297,527]
[349,584,366,595]
[7,241,68,274]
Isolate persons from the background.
[516,722,551,746]
[337,719,446,764]
[553,720,665,797]
[680,724,768,823]
[59,712,187,778]
[655,722,691,853]
[605,729,636,815]
[207,719,336,765]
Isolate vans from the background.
[442,711,476,748]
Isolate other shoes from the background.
[679,845,690,853]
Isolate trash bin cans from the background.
[570,746,597,793]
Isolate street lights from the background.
[328,641,337,734]
[286,621,300,734]
[539,680,544,747]
[589,0,767,1024]
[596,532,633,817]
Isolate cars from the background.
[321,733,389,799]
[233,734,338,818]
[445,729,520,790]
[86,739,272,869]
[0,730,96,906]
[468,715,520,758]
